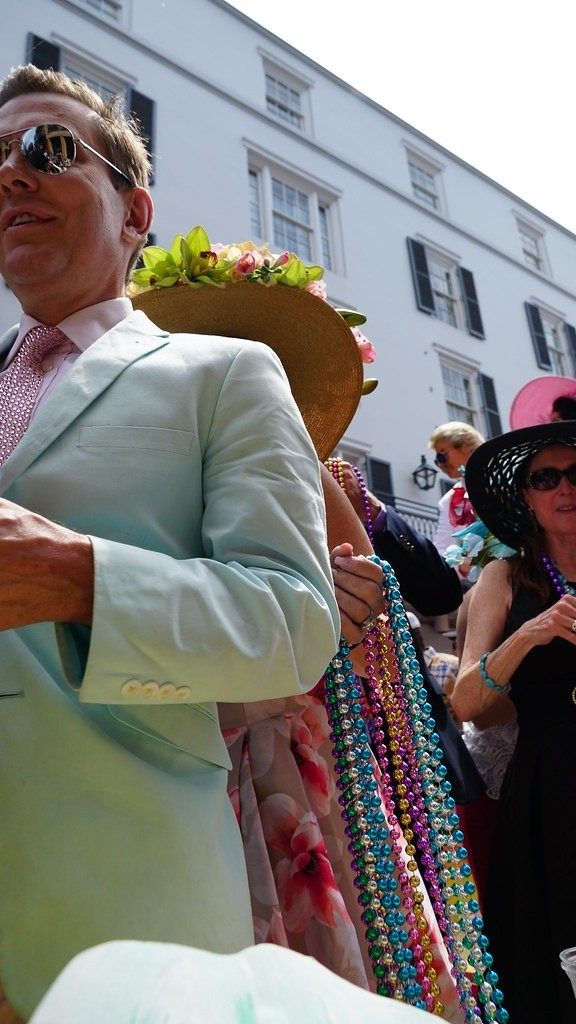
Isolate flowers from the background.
[125,227,378,396]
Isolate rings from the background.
[361,610,375,631]
[571,620,576,632]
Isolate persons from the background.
[450,420,576,1024]
[510,376,576,430]
[0,66,341,1024]
[428,422,486,581]
[324,458,488,808]
[127,226,478,1024]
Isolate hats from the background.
[510,376,576,430]
[465,419,576,552]
[126,225,378,465]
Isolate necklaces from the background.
[324,554,506,1024]
[542,554,564,599]
[351,464,374,547]
[328,458,346,492]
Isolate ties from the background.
[0,325,69,468]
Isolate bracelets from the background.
[480,652,509,691]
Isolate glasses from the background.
[434,445,457,466]
[0,124,135,188]
[522,463,576,490]
